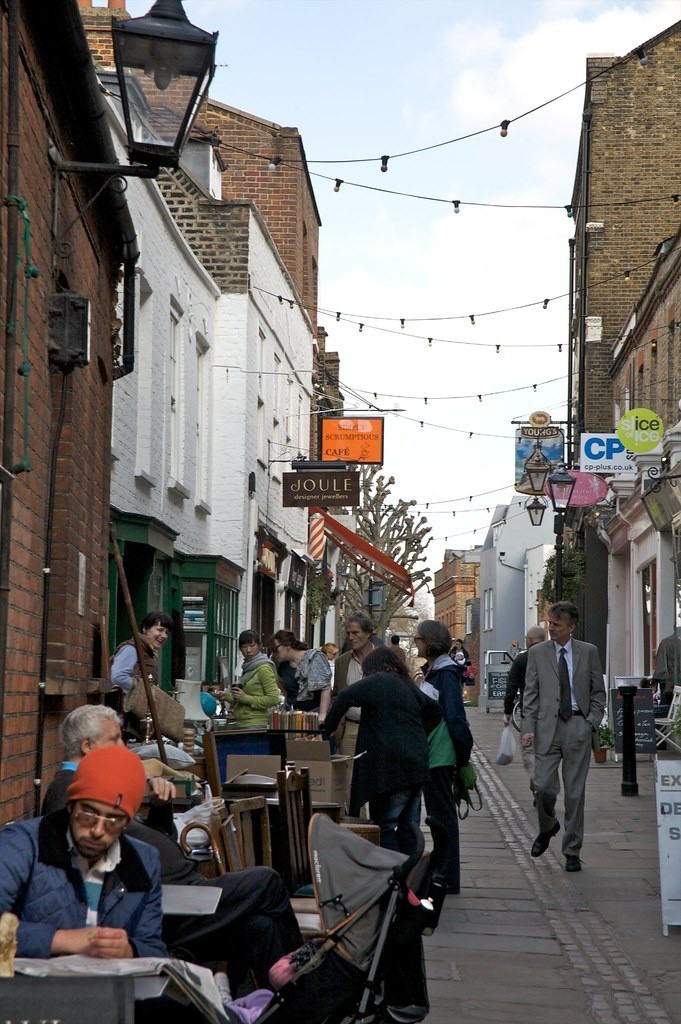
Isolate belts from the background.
[572,710,582,716]
[345,717,359,725]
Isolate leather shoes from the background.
[564,853,582,872]
[530,822,560,857]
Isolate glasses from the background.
[329,652,337,657]
[273,643,283,653]
[414,636,422,643]
[74,810,123,836]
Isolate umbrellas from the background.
[180,822,257,1001]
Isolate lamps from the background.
[584,496,616,528]
[45,0,219,177]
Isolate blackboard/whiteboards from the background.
[610,687,657,754]
[485,664,513,708]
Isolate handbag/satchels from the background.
[463,664,478,686]
[455,763,483,820]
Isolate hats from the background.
[68,745,146,825]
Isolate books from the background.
[267,707,319,739]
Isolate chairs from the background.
[211,766,322,940]
[654,685,681,752]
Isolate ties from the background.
[559,649,572,723]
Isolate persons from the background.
[1,592,297,1024]
[388,636,407,662]
[640,677,660,705]
[317,643,338,661]
[331,611,377,822]
[504,625,546,805]
[414,620,473,894]
[321,645,444,870]
[269,630,331,724]
[520,601,606,871]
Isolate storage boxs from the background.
[227,740,368,816]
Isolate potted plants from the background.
[593,725,615,763]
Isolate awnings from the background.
[308,506,415,607]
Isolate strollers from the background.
[212,808,443,1024]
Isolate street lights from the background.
[547,457,577,609]
